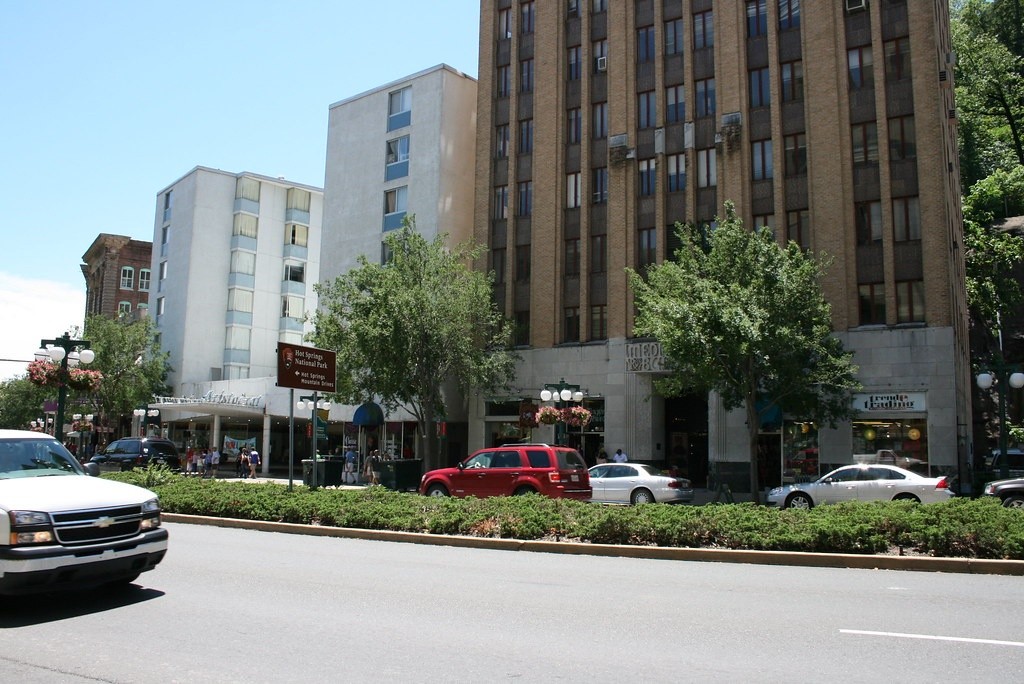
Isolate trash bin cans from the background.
[380,458,423,491]
[302,458,345,488]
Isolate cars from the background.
[588,463,696,505]
[786,448,819,474]
[988,449,1024,479]
[984,475,1024,509]
[766,462,955,512]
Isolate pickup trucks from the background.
[853,449,923,471]
[0,429,169,603]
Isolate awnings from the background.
[67,429,89,439]
[352,401,385,426]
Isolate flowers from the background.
[559,405,592,429]
[30,426,54,436]
[534,405,560,425]
[71,420,94,432]
[25,360,64,387]
[67,368,104,392]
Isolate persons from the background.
[364,449,392,484]
[233,444,261,478]
[404,442,413,459]
[186,445,220,479]
[65,441,107,465]
[613,449,628,463]
[344,448,357,484]
[310,448,340,459]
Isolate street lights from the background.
[133,407,161,437]
[540,377,583,446]
[976,364,1024,479]
[72,414,94,457]
[34,332,95,444]
[31,418,53,433]
[297,390,332,487]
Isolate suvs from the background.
[419,443,594,503]
[90,436,183,475]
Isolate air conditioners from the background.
[846,0,867,15]
[947,107,959,125]
[939,69,951,88]
[944,52,956,67]
